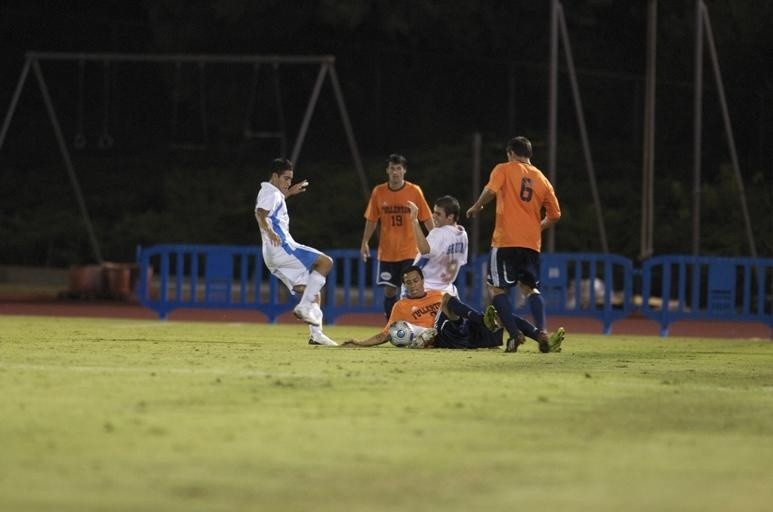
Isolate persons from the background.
[341,266,565,352]
[253,157,339,346]
[399,195,469,301]
[359,153,435,324]
[464,136,562,353]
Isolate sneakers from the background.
[483,305,498,334]
[548,326,567,354]
[292,304,320,327]
[307,334,339,348]
[502,330,527,353]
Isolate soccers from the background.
[387,320,414,347]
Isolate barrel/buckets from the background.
[569,275,605,310]
[67,263,103,298]
[103,260,153,300]
